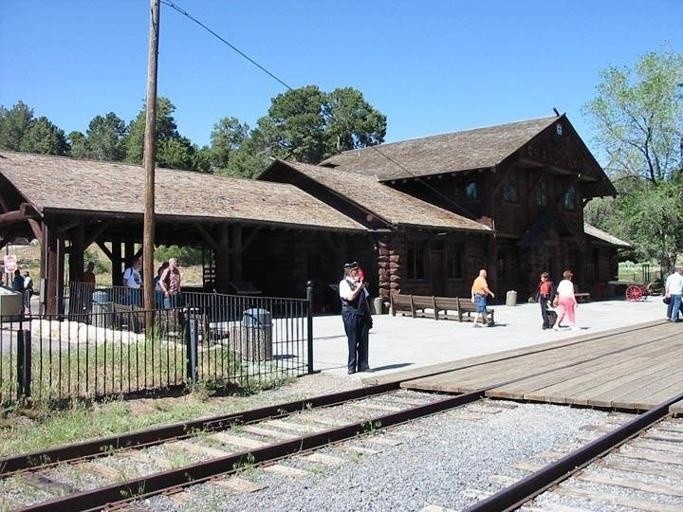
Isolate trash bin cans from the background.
[92,291,109,327]
[240,308,273,362]
[505,290,518,306]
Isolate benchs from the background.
[390,291,494,323]
[112,301,229,344]
[573,284,590,302]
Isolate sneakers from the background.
[472,321,495,329]
[347,368,374,375]
[541,324,582,332]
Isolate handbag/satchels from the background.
[551,294,560,307]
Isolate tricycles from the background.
[620,261,673,303]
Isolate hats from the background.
[562,270,573,277]
[342,261,359,268]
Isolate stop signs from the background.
[4,255,18,273]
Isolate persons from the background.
[470,269,494,328]
[77,261,95,313]
[159,257,180,309]
[551,271,578,330]
[22,271,33,314]
[338,262,371,375]
[534,272,557,330]
[122,259,142,305]
[664,270,682,322]
[12,269,24,290]
[153,267,163,290]
